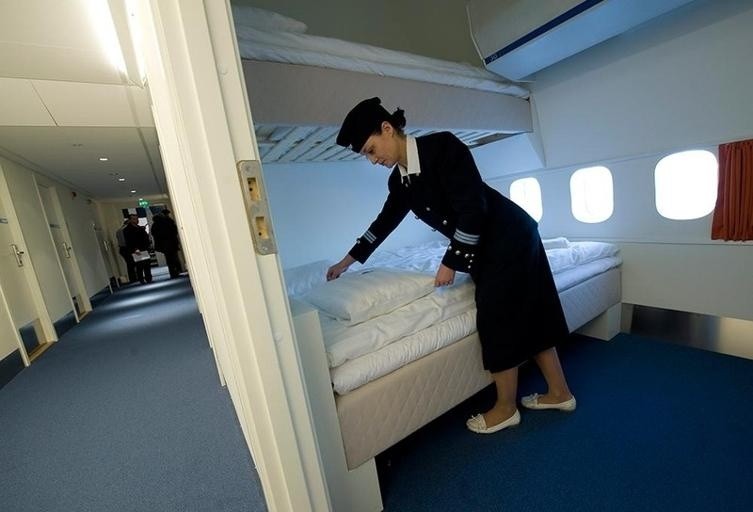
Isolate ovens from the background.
[288,242,620,512]
[230,0,534,163]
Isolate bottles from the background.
[336,97,388,152]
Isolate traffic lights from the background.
[284,261,329,296]
[303,268,437,327]
[233,5,308,36]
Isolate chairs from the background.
[466,409,521,434]
[521,393,576,413]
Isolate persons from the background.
[326,97,576,435]
[116,210,182,284]
